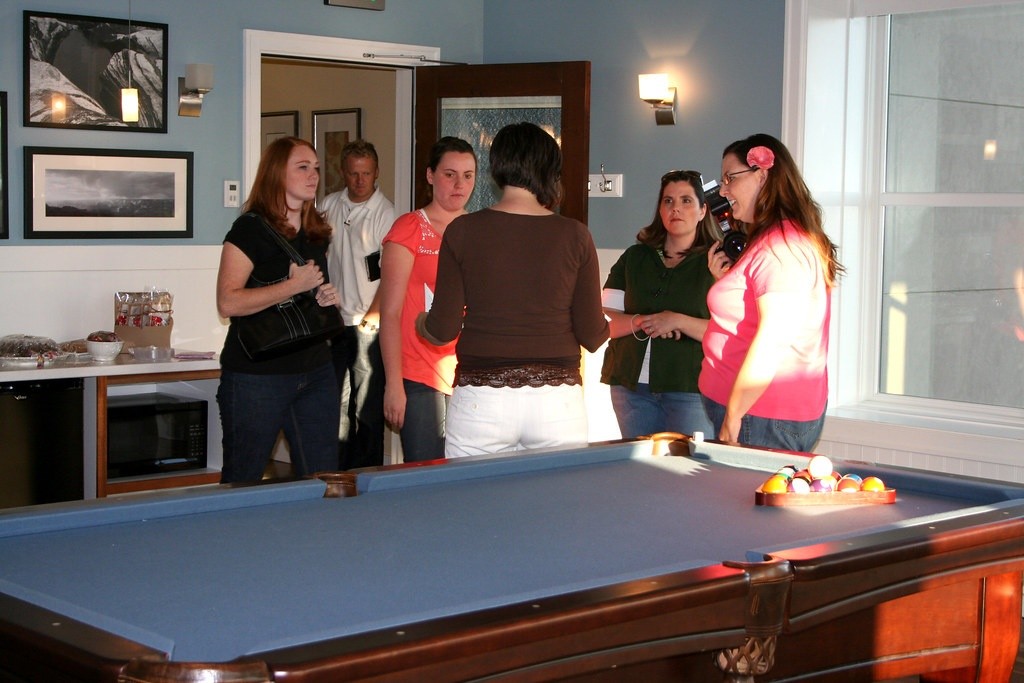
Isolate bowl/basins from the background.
[86,340,124,362]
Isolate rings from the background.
[650,327,655,333]
[332,293,336,299]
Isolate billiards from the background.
[760,455,885,492]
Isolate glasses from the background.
[721,169,751,185]
[661,170,704,185]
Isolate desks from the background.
[0,430,1024,683]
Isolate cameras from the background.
[702,180,747,261]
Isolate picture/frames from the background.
[23,10,169,134]
[311,107,361,206]
[261,110,300,160]
[23,146,194,238]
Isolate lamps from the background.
[120,0,138,122]
[639,73,677,125]
[177,63,215,117]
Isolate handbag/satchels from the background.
[234,213,344,362]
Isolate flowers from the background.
[747,146,775,170]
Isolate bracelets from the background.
[359,319,380,332]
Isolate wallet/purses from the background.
[365,251,382,282]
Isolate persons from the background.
[599,169,721,441]
[216,138,347,479]
[415,122,611,455]
[314,138,401,465]
[381,134,481,461]
[696,133,847,451]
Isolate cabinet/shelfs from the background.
[0,355,229,499]
